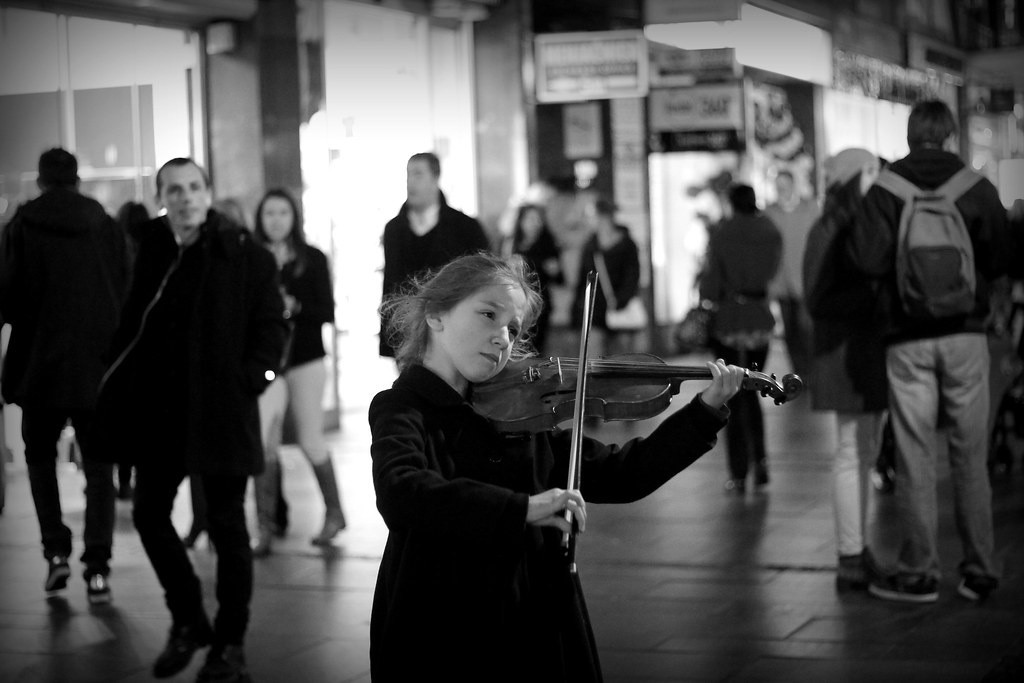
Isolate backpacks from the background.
[875,167,987,316]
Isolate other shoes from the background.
[724,466,769,492]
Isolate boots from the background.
[313,457,346,545]
[252,462,279,559]
[152,587,212,679]
[195,610,248,683]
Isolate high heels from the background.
[183,493,212,549]
[276,493,287,538]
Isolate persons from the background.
[0,100,1024,683]
[365,249,747,683]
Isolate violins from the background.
[468,352,802,439]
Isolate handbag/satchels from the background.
[675,306,713,350]
[604,293,649,332]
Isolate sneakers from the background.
[837,551,881,592]
[44,556,71,591]
[869,571,941,601]
[957,576,984,600]
[83,566,111,603]
